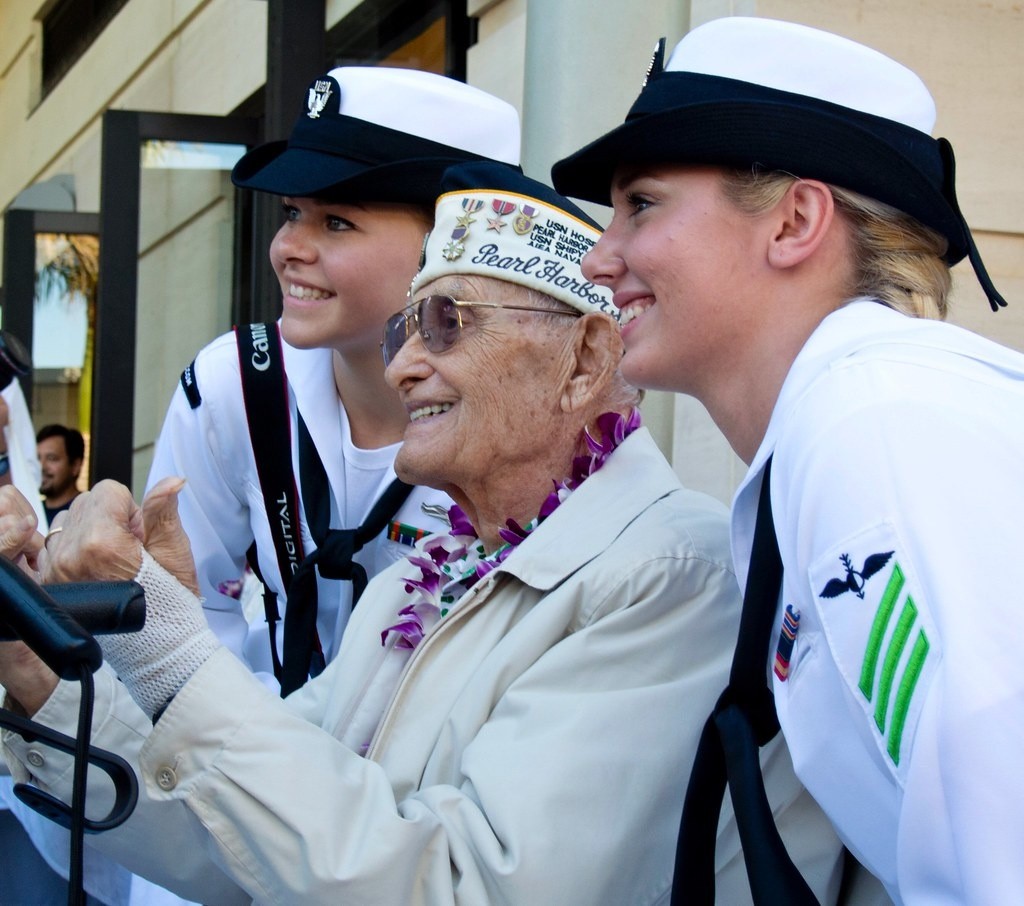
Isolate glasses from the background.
[378,294,582,366]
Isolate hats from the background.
[551,17,1009,312]
[230,67,521,205]
[409,162,621,324]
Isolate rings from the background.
[44,528,63,549]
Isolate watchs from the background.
[0,450,10,476]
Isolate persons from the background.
[544,14,1022,906]
[0,172,845,906]
[0,391,85,529]
[137,63,525,696]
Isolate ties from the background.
[669,455,818,906]
[278,410,416,700]
